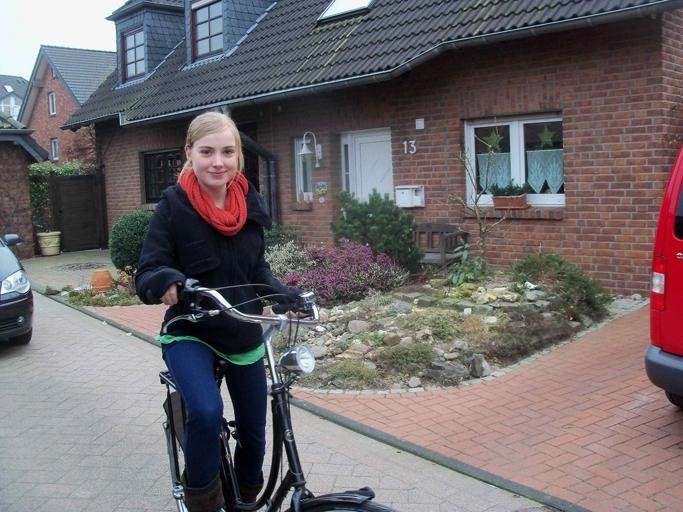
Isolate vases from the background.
[36,230,63,256]
[91,268,116,293]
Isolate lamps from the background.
[298,131,321,168]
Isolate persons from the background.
[133,109,313,512]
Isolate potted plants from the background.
[489,182,531,210]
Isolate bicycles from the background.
[159,277,395,512]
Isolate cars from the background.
[645,146,683,408]
[0,234,33,346]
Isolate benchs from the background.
[413,224,469,266]
